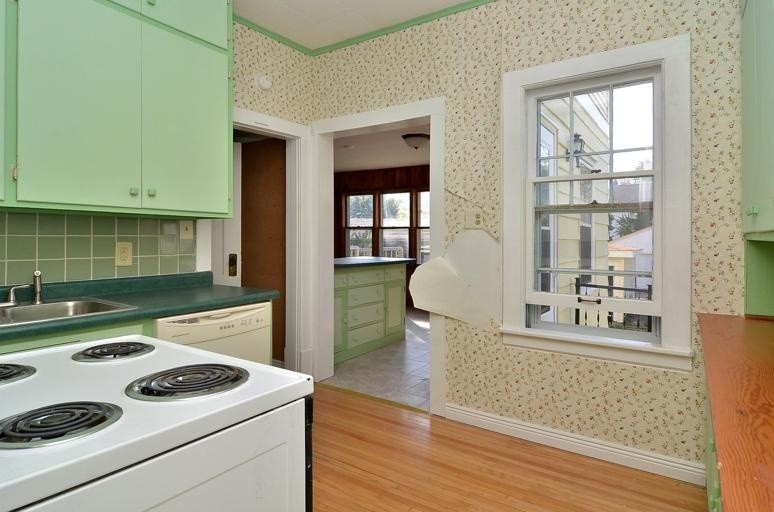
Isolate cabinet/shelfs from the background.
[696,3,774,512]
[333,262,409,365]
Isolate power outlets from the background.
[114,241,132,266]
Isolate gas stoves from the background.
[0,334,313,511]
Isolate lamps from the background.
[401,133,429,150]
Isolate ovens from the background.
[153,301,273,367]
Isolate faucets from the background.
[32,271,44,304]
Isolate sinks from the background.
[0,296,141,330]
